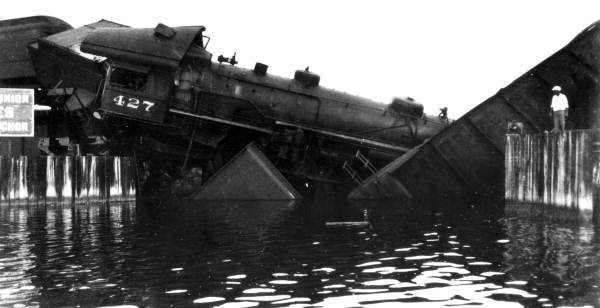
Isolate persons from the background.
[550,86,568,131]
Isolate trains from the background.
[0,16,599,206]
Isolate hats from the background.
[552,85,562,91]
